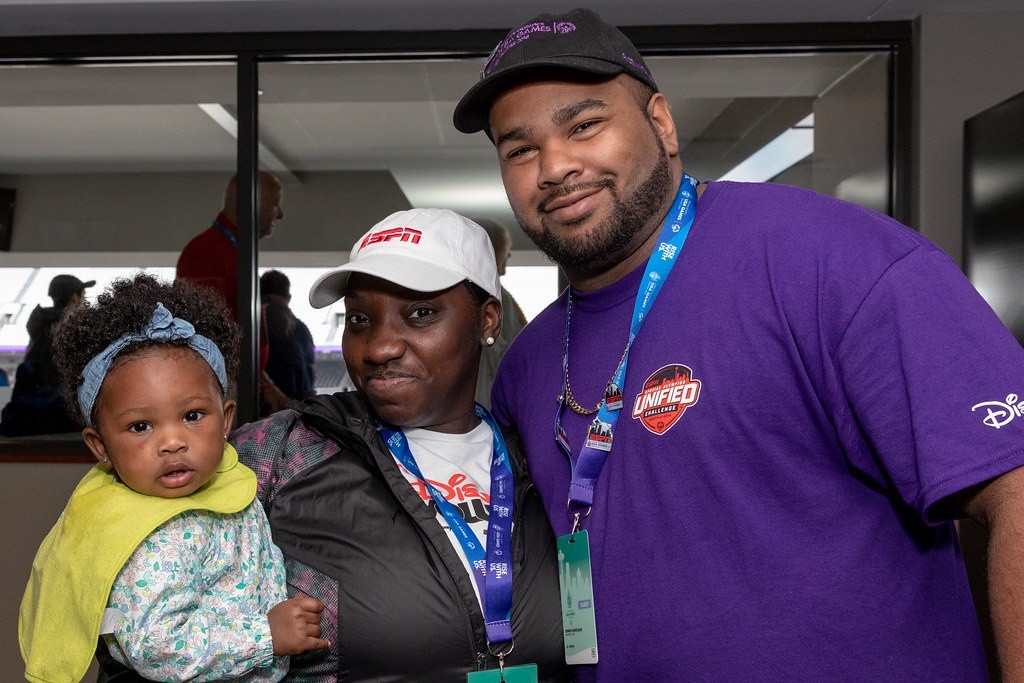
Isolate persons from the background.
[464,215,529,415]
[224,207,598,683]
[11,275,96,438]
[17,276,330,683]
[174,169,290,416]
[454,7,1024,683]
[259,270,317,411]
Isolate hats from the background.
[453,9,657,135]
[309,208,502,308]
[259,271,291,295]
[49,275,96,301]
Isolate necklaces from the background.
[561,291,632,414]
